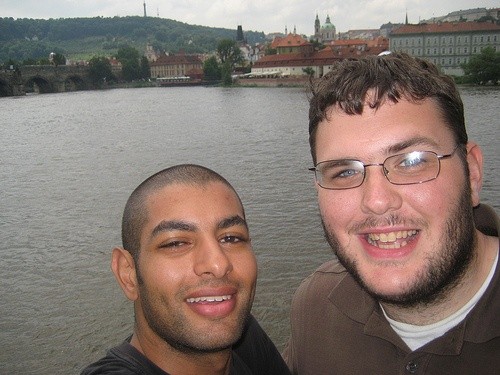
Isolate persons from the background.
[287,50,500,375]
[79,164,293,375]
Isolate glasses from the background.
[308,143,463,190]
